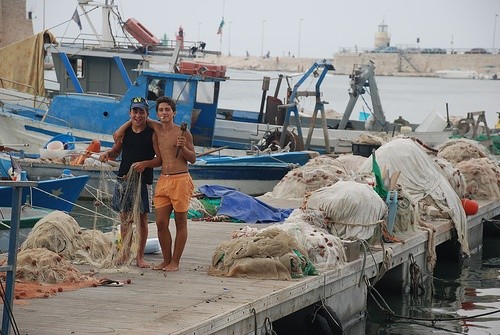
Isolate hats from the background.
[130,97,149,111]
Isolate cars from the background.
[462,47,491,54]
[367,45,447,54]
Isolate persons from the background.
[112,97,197,271]
[99,97,163,269]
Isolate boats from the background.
[0,157,302,203]
[476,64,498,79]
[0,167,91,254]
[0,0,457,158]
[26,0,223,73]
[434,65,477,79]
